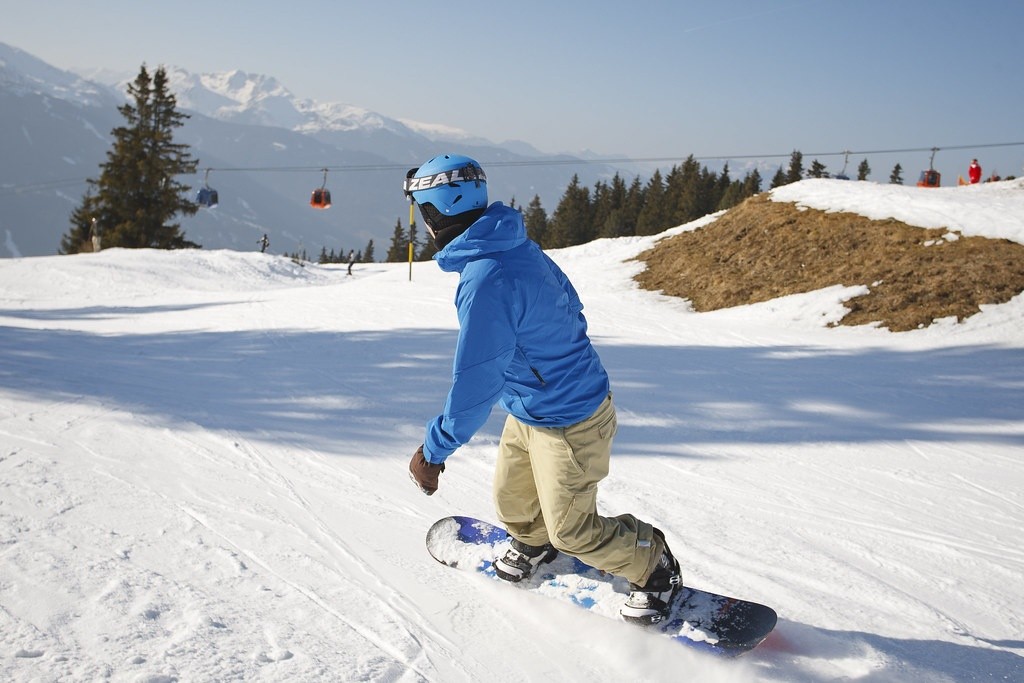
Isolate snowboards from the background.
[426,518,777,657]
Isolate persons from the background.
[404,154,682,625]
[968,159,981,183]
[256,234,269,253]
[89,217,103,252]
[346,249,355,275]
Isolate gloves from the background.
[408,446,446,497]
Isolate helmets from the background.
[405,156,486,231]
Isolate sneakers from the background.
[620,549,683,624]
[495,538,560,582]
